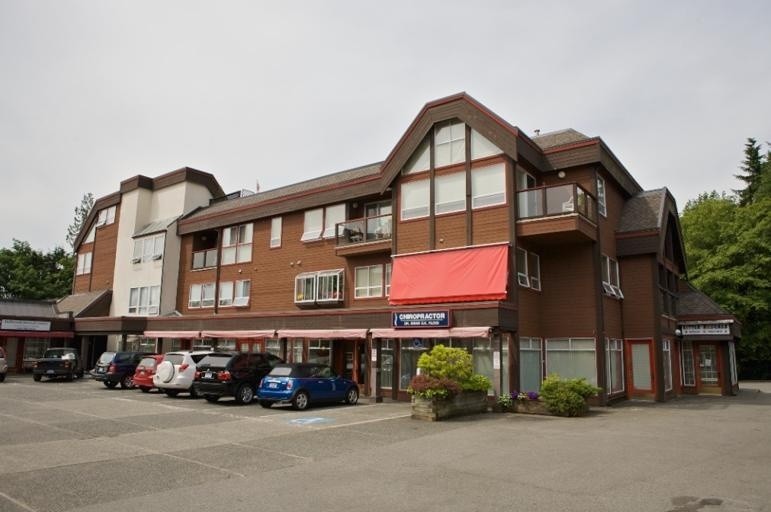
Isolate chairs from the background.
[563,195,575,212]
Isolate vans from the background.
[22,345,44,371]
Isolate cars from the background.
[302,353,416,389]
[0,347,8,382]
[94,349,359,411]
[32,347,84,381]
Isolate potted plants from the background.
[497,376,602,419]
[408,344,489,422]
